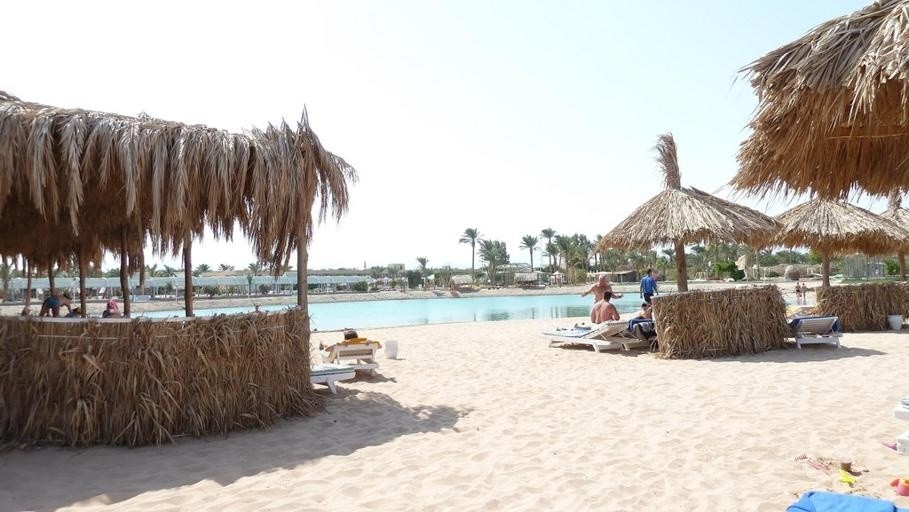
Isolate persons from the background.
[794,283,809,300]
[320,329,356,351]
[23,292,120,317]
[582,269,659,325]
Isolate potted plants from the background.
[887,305,904,330]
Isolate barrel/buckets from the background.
[888,315,902,330]
[384,340,397,359]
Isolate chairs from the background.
[541,320,658,353]
[309,342,381,394]
[788,315,843,349]
